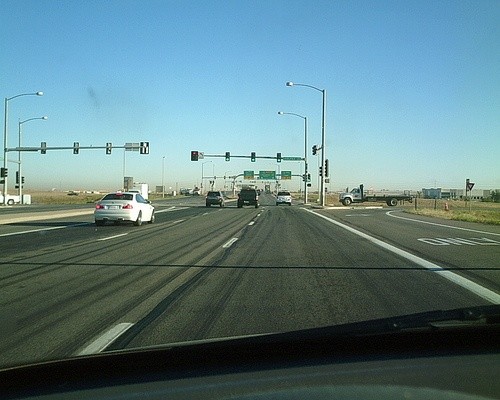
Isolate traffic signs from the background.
[259,171,275,179]
[281,171,291,179]
[467,183,475,190]
[244,171,254,179]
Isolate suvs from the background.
[275,190,292,206]
[206,191,225,208]
[193,189,200,196]
[237,189,259,208]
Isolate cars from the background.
[94,192,155,226]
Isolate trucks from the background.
[339,184,413,206]
[180,188,193,195]
[67,190,77,195]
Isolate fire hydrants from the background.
[445,203,449,210]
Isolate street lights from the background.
[162,156,165,198]
[286,81,326,207]
[200,160,212,195]
[278,111,309,204]
[18,116,47,205]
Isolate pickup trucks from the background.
[0,191,19,205]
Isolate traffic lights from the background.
[1,168,8,177]
[251,152,256,162]
[191,151,198,161]
[312,145,316,155]
[319,167,322,176]
[226,152,230,161]
[308,174,310,179]
[4,91,44,205]
[302,174,305,181]
[22,177,24,183]
[325,159,329,177]
[277,153,281,162]
